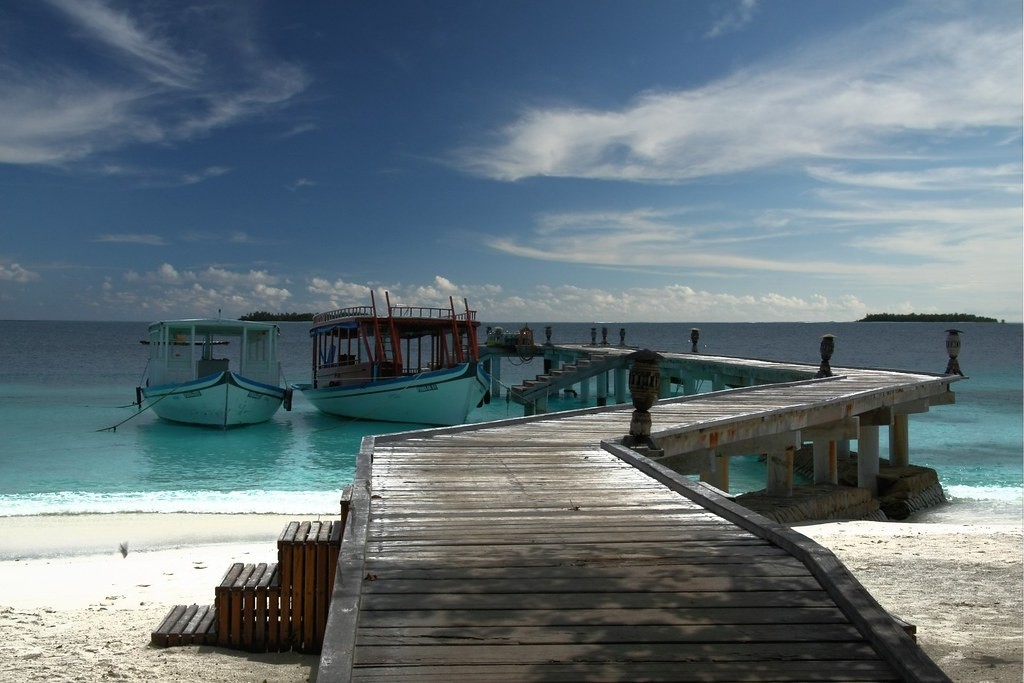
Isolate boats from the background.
[138,317,291,429]
[291,290,490,428]
